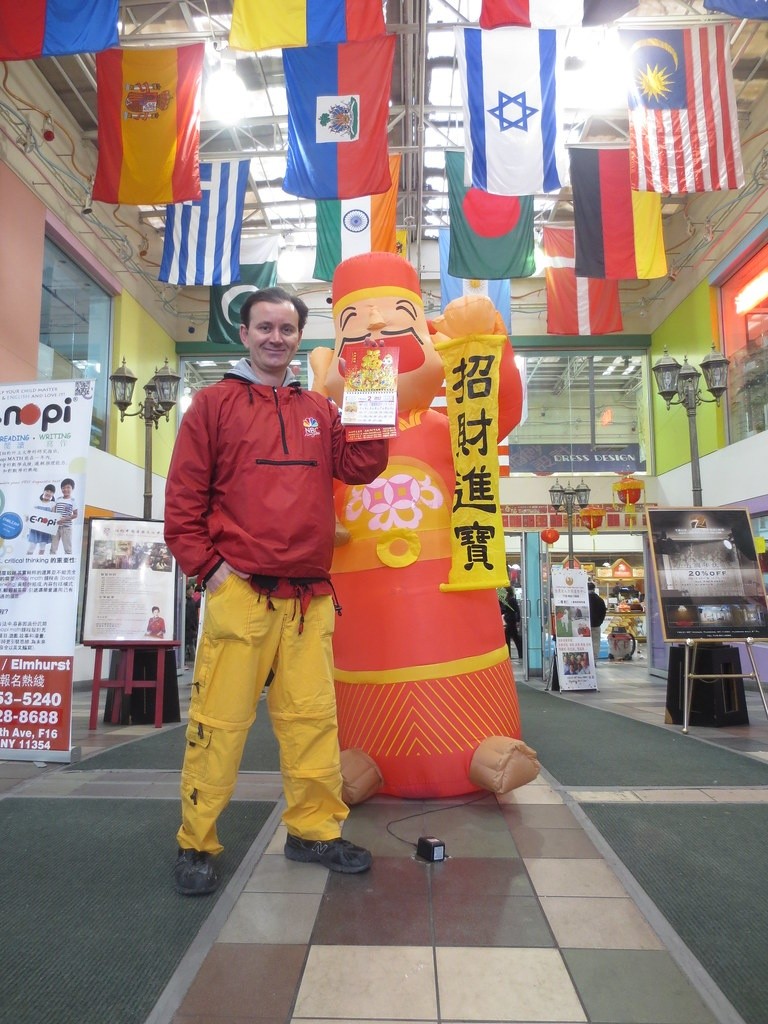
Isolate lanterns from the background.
[580,506,606,551]
[542,527,559,548]
[612,477,644,531]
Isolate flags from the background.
[0,0,407,284]
[565,141,666,279]
[444,149,536,281]
[439,227,511,335]
[207,236,279,344]
[479,0,640,31]
[543,224,623,336]
[619,21,745,193]
[452,25,564,196]
[703,0,768,20]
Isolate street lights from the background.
[108,356,182,520]
[648,339,732,512]
[548,476,592,571]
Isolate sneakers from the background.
[173,848,218,895]
[283,832,372,874]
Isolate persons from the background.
[564,653,590,675]
[499,587,522,658]
[588,583,606,668]
[27,479,78,555]
[165,287,390,895]
[147,606,165,638]
[307,252,540,803]
[578,622,590,637]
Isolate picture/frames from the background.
[645,505,768,643]
[79,516,178,644]
